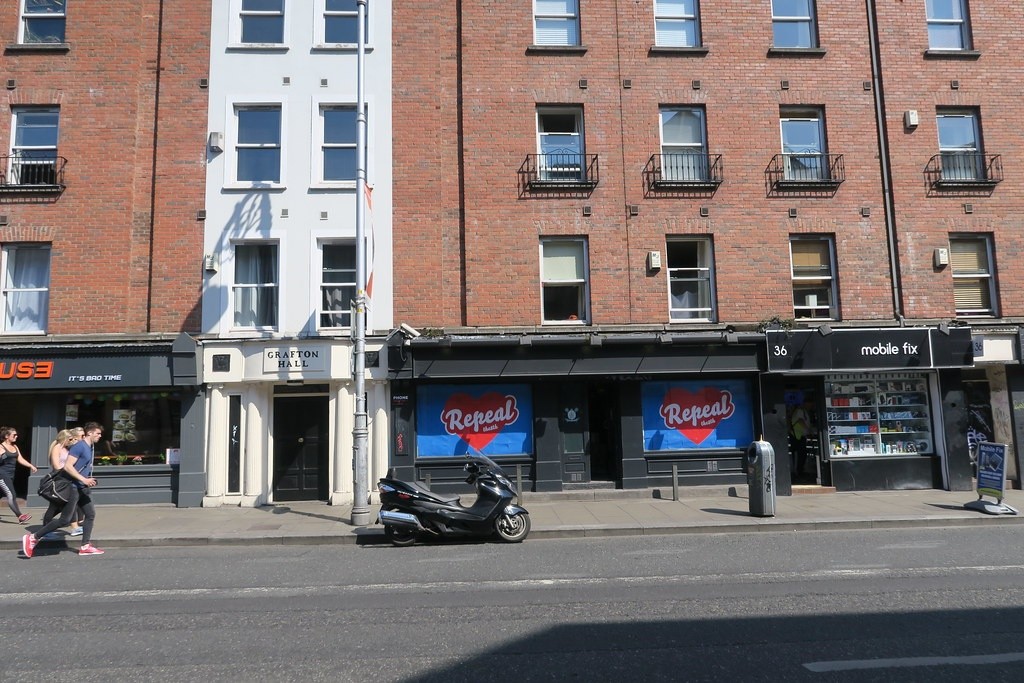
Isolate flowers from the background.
[133,456,142,462]
[102,456,110,461]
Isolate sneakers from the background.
[18,513,32,525]
[71,527,84,536]
[23,533,38,558]
[79,543,105,555]
[42,532,59,539]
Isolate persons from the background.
[789,396,812,477]
[0,426,37,524]
[23,422,105,558]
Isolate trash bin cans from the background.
[748,441,776,517]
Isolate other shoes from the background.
[79,520,83,525]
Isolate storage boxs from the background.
[840,412,870,420]
[841,386,849,394]
[826,397,858,406]
[857,426,869,433]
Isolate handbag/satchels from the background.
[37,470,73,505]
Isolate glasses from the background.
[81,435,85,439]
[69,439,73,441]
[12,434,17,438]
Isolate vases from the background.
[104,461,109,465]
[135,462,143,464]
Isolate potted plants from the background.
[115,453,127,465]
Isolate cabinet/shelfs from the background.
[824,378,934,457]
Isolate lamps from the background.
[818,324,833,337]
[590,332,602,348]
[520,332,532,347]
[725,330,738,345]
[660,332,673,344]
[438,334,451,348]
[937,324,950,335]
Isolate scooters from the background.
[372,443,533,547]
